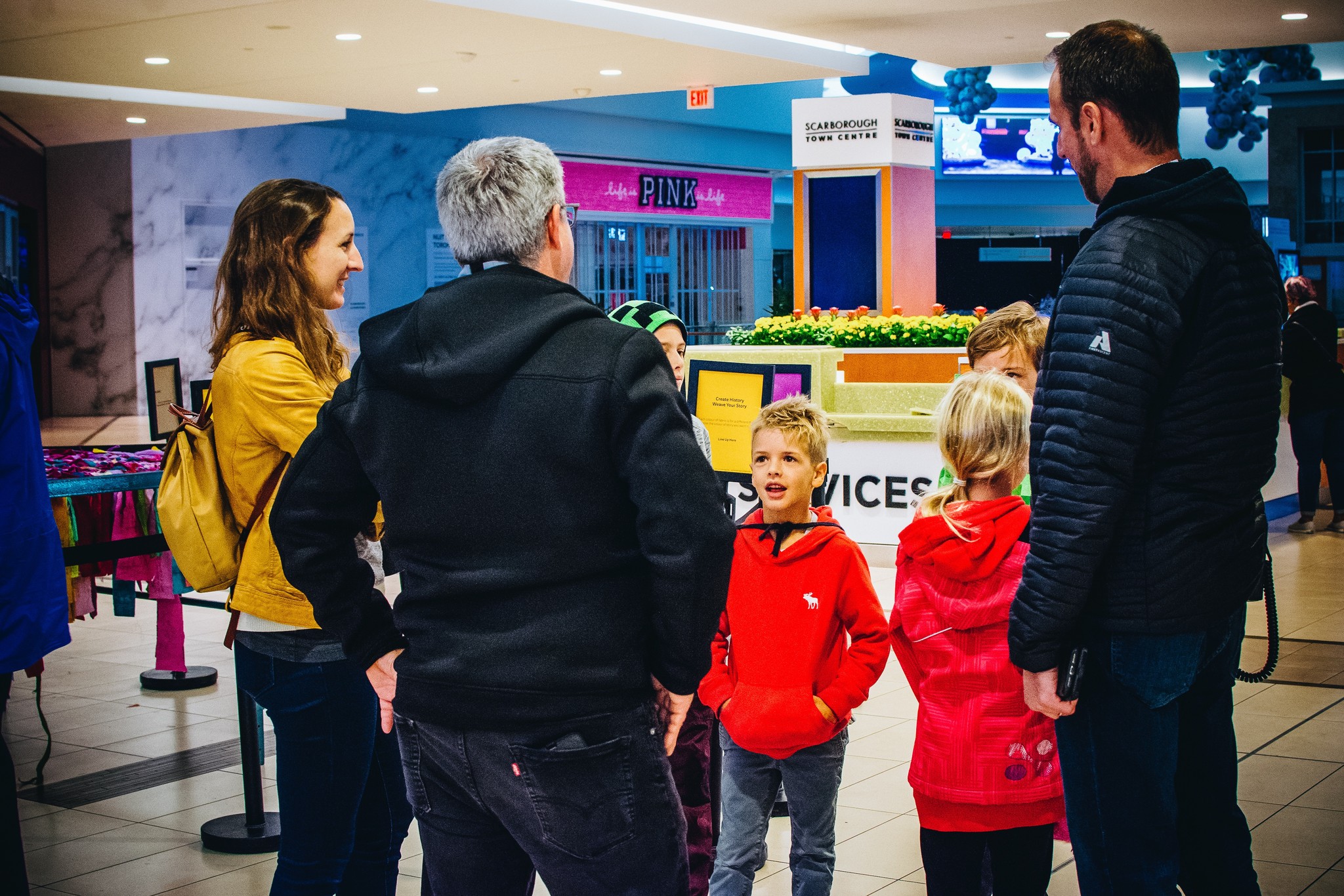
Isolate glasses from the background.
[545,203,580,228]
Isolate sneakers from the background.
[1288,520,1315,534]
[1327,520,1344,532]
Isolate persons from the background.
[1278,275,1344,534]
[268,137,733,896]
[1006,19,1285,896]
[889,369,1071,896]
[700,388,891,896]
[937,302,1051,506]
[0,268,71,896]
[205,178,413,896]
[1050,132,1064,175]
[601,300,720,896]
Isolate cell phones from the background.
[1056,646,1089,703]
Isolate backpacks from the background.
[156,337,291,594]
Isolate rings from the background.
[1058,712,1063,716]
